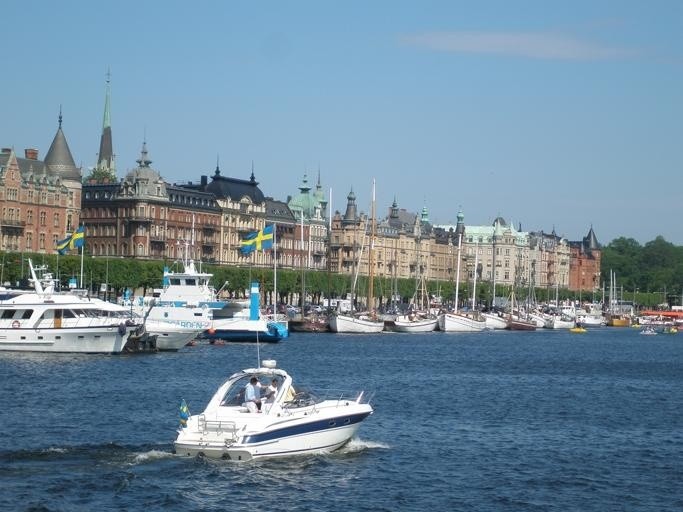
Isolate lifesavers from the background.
[12,320,20,328]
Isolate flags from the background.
[240,224,274,253]
[56,225,85,255]
[179,399,191,425]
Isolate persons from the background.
[244,377,264,413]
[264,378,279,402]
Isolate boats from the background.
[173,359,374,463]
